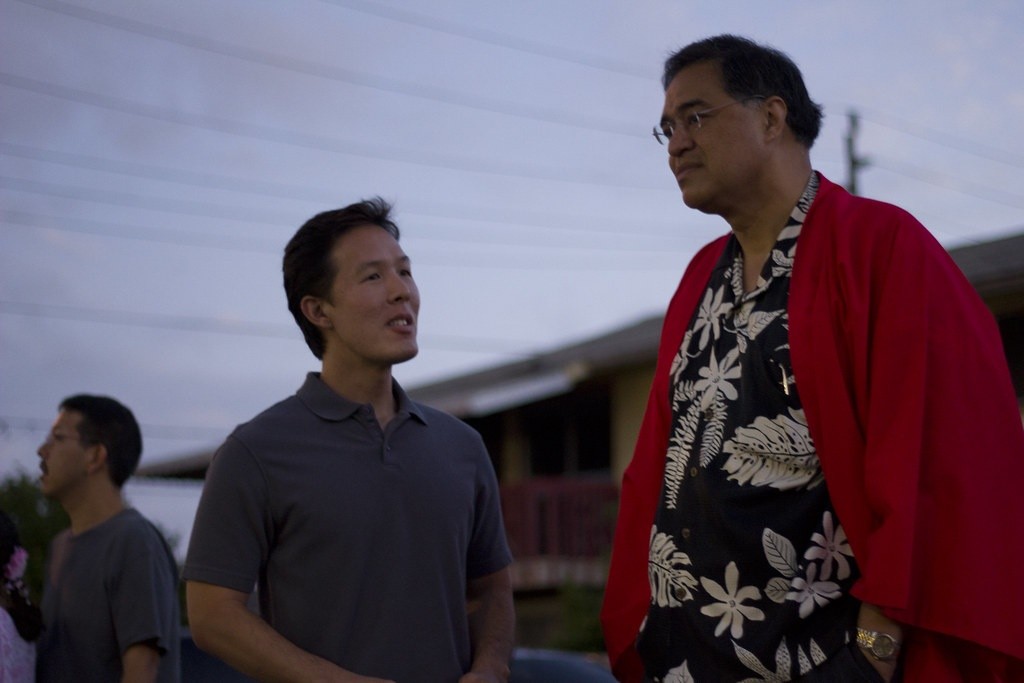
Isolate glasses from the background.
[47,433,81,446]
[652,94,767,145]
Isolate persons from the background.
[0,393,178,682]
[600,35,1024,683]
[177,200,517,683]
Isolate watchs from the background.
[856,625,901,661]
